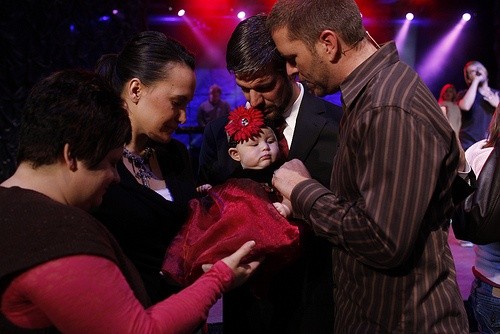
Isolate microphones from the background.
[476,71,481,76]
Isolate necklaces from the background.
[122,145,160,189]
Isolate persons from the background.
[458,62,500,247]
[437,84,461,133]
[99,30,223,334]
[272,0,469,334]
[451,104,500,334]
[0,70,259,334]
[196,85,231,128]
[195,105,293,271]
[201,15,344,334]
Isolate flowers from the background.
[224,105,265,142]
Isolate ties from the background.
[268,118,289,154]
